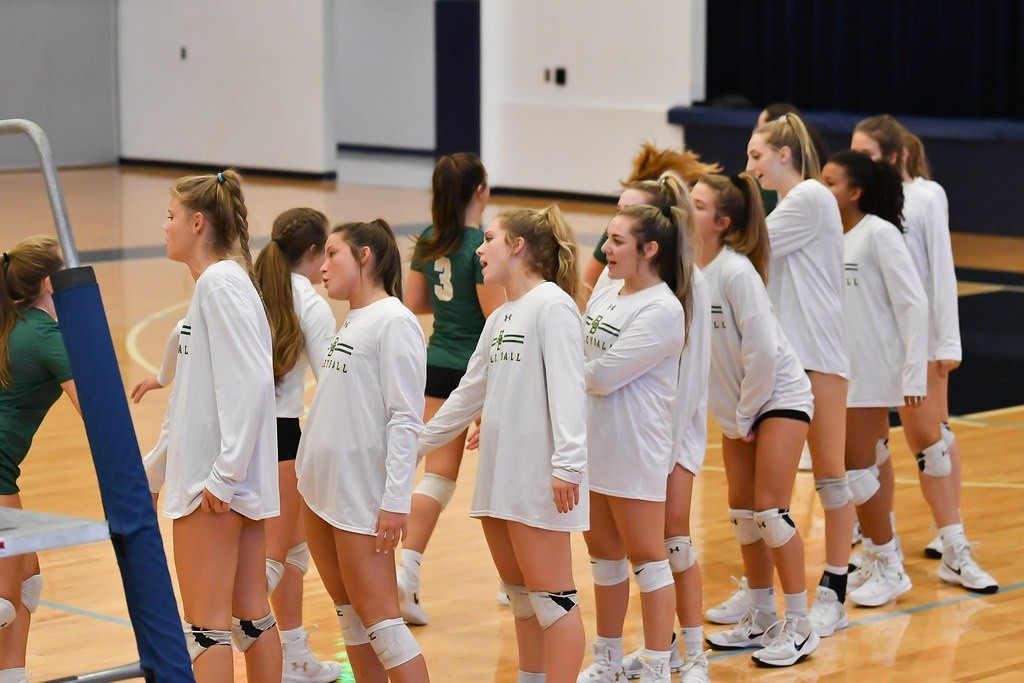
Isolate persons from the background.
[851,115,1000,595]
[851,117,982,560]
[819,147,930,606]
[703,111,849,638]
[691,171,820,669]
[581,142,722,311]
[416,202,591,683]
[616,170,712,683]
[575,205,693,683]
[0,235,84,683]
[295,218,430,683]
[131,207,342,683]
[395,152,511,626]
[143,167,283,683]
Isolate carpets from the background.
[888,290,1024,429]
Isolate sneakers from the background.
[394,563,429,626]
[575,642,628,683]
[639,649,672,683]
[848,551,913,607]
[807,585,849,637]
[924,533,944,559]
[936,542,999,594]
[622,631,684,678]
[750,616,821,667]
[679,649,717,683]
[705,611,782,651]
[280,631,341,683]
[703,574,753,624]
[848,519,863,547]
[845,553,871,593]
